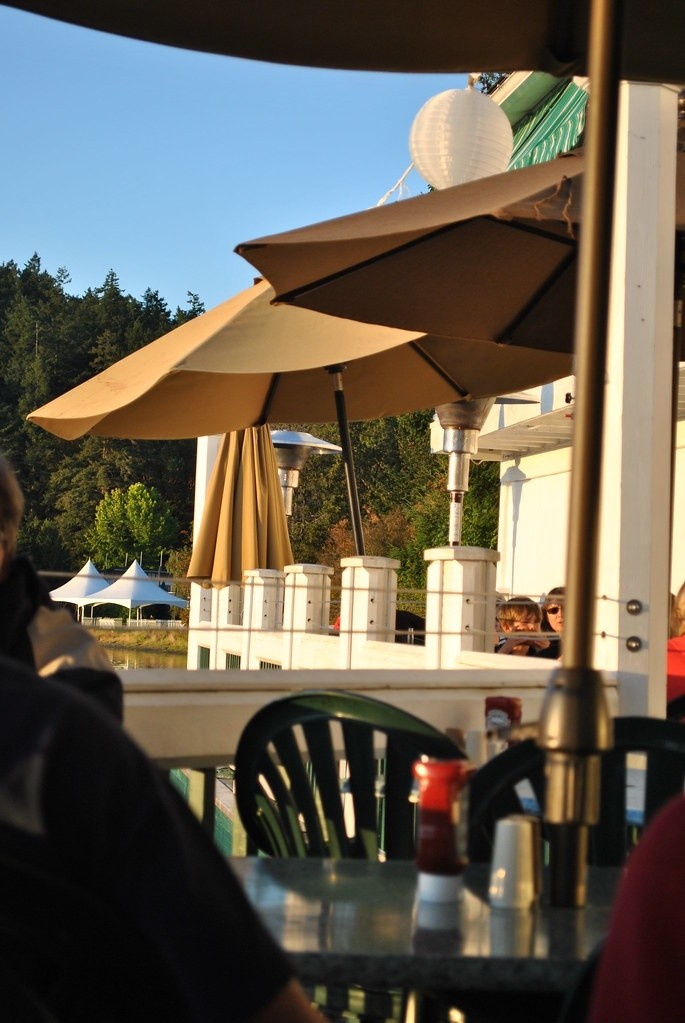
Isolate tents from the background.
[48,557,190,626]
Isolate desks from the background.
[220,855,626,994]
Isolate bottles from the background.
[413,758,468,903]
[489,819,534,909]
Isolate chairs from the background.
[233,687,685,1023]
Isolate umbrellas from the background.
[187,424,295,589]
[0,0,685,820]
[232,144,685,360]
[27,276,579,555]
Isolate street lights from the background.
[436,392,540,548]
[271,429,341,520]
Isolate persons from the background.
[0,654,330,1023]
[495,587,566,660]
[0,453,123,726]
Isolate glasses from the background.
[546,607,563,615]
